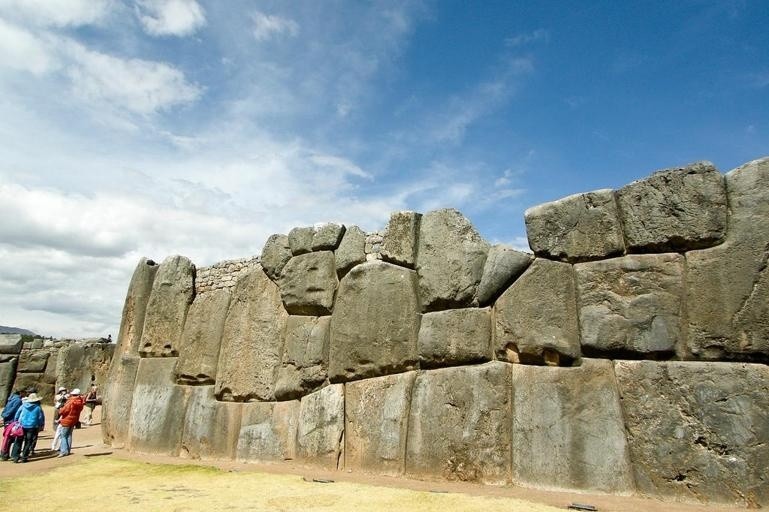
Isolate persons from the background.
[0,387,97,464]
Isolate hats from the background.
[70,388,80,394]
[58,387,67,394]
[16,386,43,402]
